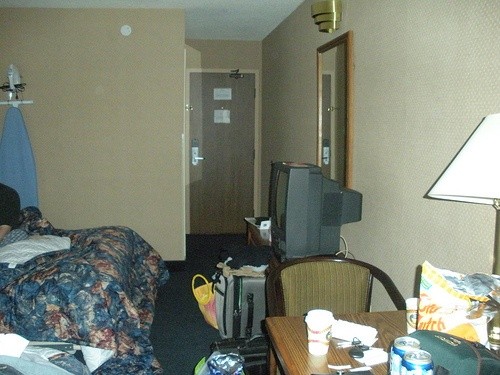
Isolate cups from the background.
[406,298,420,334]
[305,310,334,355]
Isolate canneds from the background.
[389,336,434,375]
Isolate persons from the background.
[0,183,21,241]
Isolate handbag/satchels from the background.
[418,261,500,350]
[192,274,217,329]
[207,353,245,375]
[210,336,268,375]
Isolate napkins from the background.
[332,319,378,347]
[354,347,389,367]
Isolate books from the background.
[28,341,74,350]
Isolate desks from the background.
[0,340,92,375]
[261,310,418,375]
[243,217,269,248]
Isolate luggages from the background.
[214,270,266,338]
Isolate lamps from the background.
[311,0,342,34]
[424,113,500,274]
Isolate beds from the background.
[0,205,173,375]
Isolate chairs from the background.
[266,255,406,320]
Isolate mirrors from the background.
[317,29,353,188]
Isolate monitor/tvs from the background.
[268,162,362,263]
